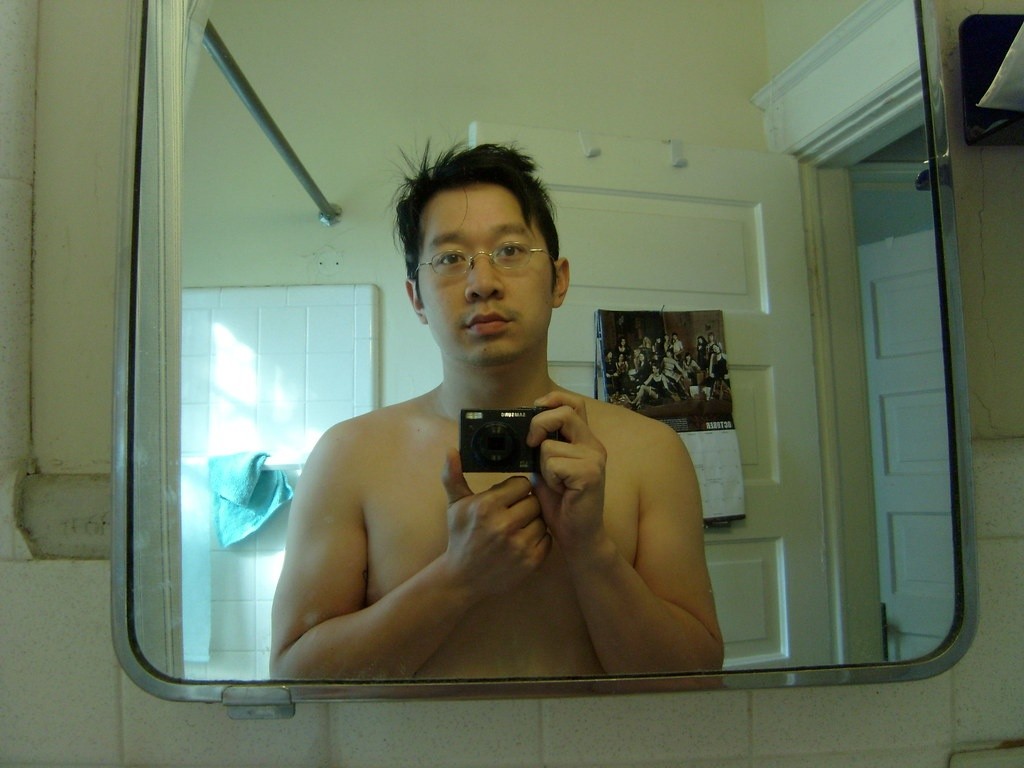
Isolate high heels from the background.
[635,403,642,410]
[628,401,636,406]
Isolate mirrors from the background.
[109,0,981,705]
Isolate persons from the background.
[271,143,723,680]
[605,332,729,408]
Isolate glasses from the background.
[414,241,554,278]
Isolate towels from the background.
[209,449,296,549]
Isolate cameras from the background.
[459,407,566,473]
